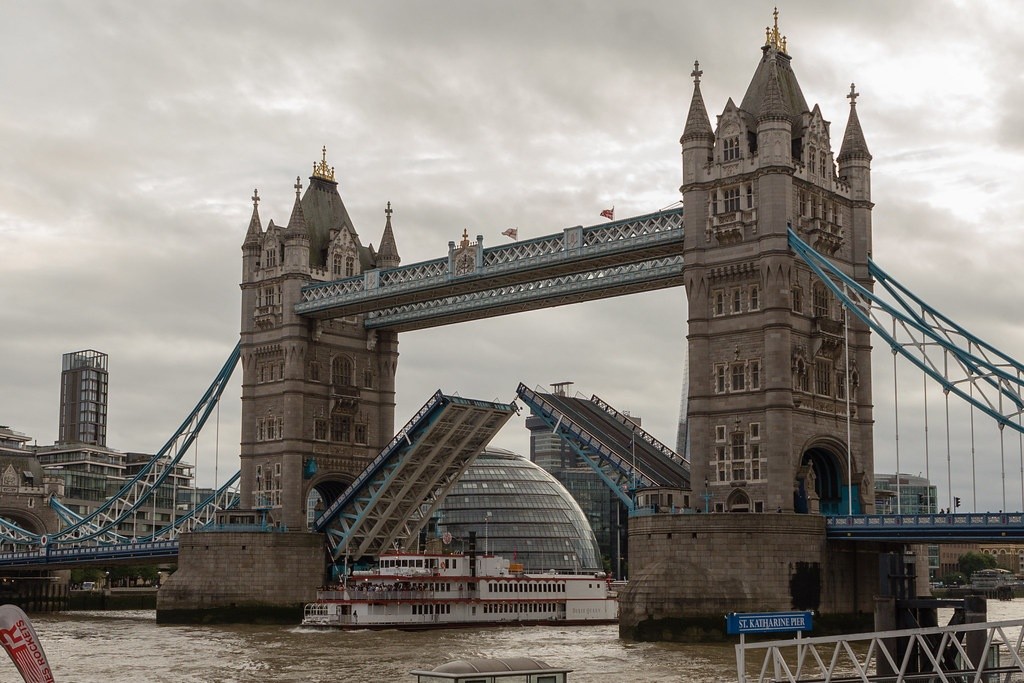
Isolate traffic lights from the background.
[956,497,960,507]
[919,494,923,503]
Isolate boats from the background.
[300,530,619,630]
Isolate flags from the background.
[502,228,516,241]
[600,209,613,220]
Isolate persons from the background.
[776,506,782,513]
[696,506,701,513]
[938,508,950,515]
[314,582,465,599]
[353,610,359,624]
[189,527,196,532]
[653,503,660,514]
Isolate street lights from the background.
[106,571,109,589]
[157,571,162,588]
